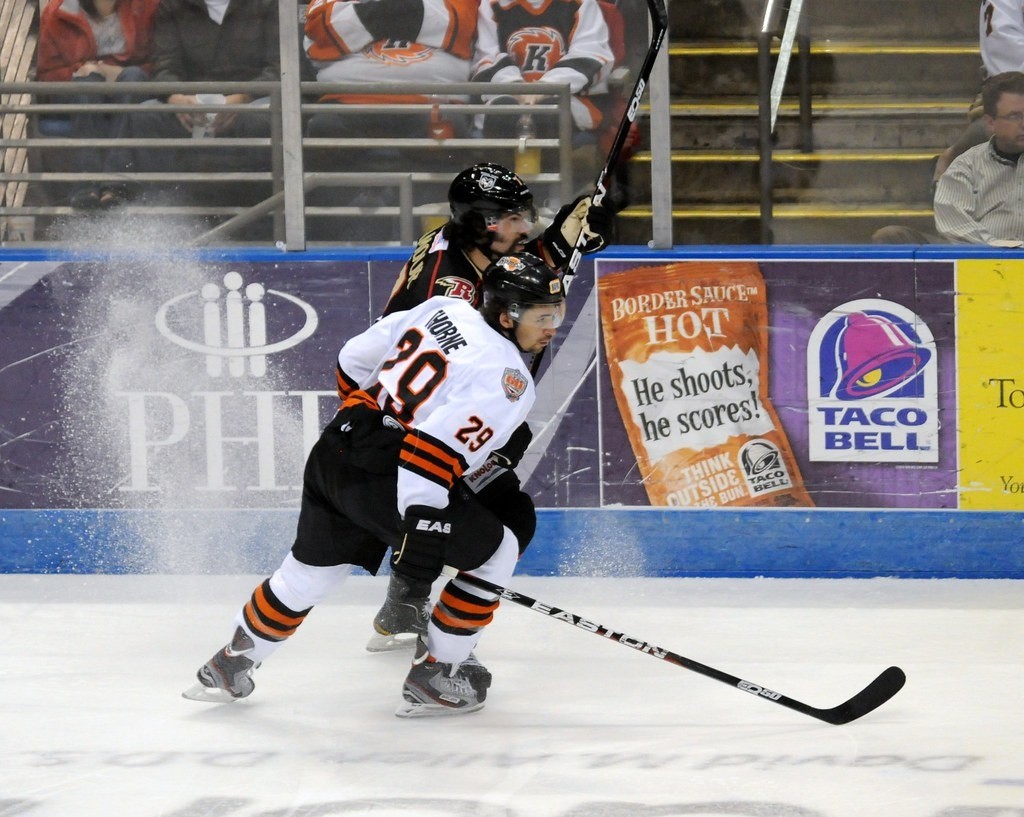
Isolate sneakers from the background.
[182,625,255,703]
[460,652,490,688]
[394,633,486,718]
[365,571,432,652]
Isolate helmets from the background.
[447,162,534,240]
[482,251,566,310]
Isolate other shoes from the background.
[69,185,121,210]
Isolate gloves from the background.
[390,504,453,588]
[544,196,615,274]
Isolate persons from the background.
[31,0,638,211]
[871,0,1024,246]
[182,163,612,717]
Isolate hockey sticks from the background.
[453,569,910,727]
[561,0,671,298]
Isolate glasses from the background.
[995,110,1024,123]
